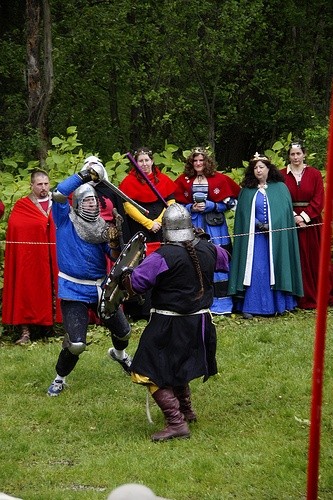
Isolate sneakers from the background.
[107,347,133,376]
[47,380,65,397]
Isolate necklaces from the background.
[195,174,204,183]
[293,170,302,177]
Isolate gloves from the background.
[259,223,269,234]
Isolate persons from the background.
[173,146,242,316]
[47,168,134,397]
[3,170,63,347]
[65,156,126,326]
[279,141,333,311]
[108,483,164,500]
[120,202,230,443]
[229,152,305,320]
[114,144,179,321]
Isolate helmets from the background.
[72,182,101,222]
[162,203,195,241]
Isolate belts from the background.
[150,308,210,316]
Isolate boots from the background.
[15,324,30,345]
[151,386,191,442]
[172,383,196,423]
